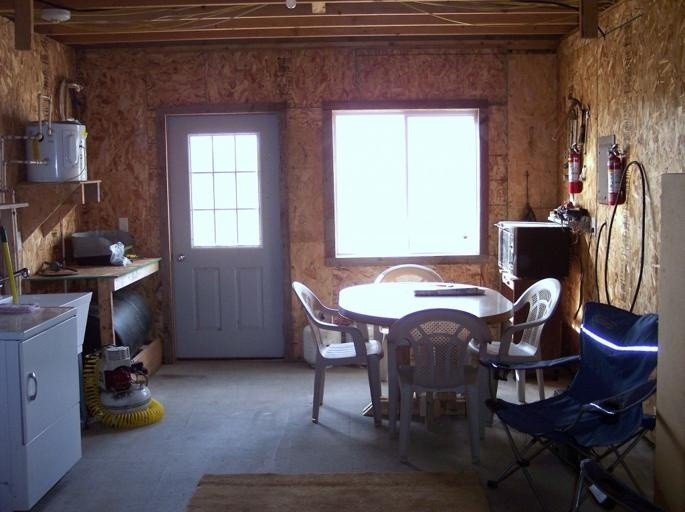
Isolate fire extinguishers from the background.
[606,143,625,205]
[567,143,583,194]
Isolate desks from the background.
[22,257,162,379]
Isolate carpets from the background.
[184,471,492,512]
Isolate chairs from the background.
[480,298,658,511]
